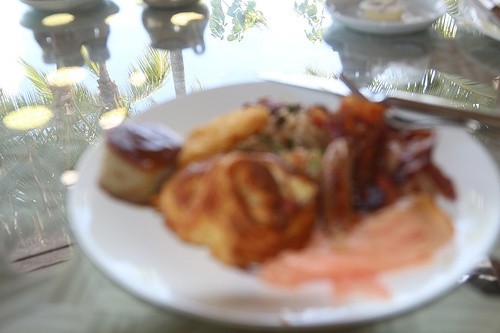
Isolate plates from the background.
[67,80,500,332]
[326,0,448,34]
[19,2,118,32]
[21,1,95,11]
[458,1,500,40]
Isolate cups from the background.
[33,21,110,65]
[145,0,199,10]
[141,5,208,55]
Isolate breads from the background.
[157,153,320,270]
[175,106,272,166]
